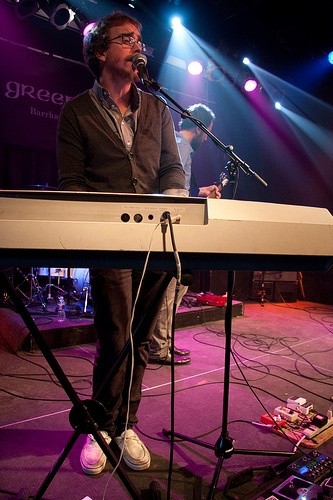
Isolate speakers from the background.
[250,272,306,301]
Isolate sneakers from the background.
[113,427,151,471]
[80,430,111,475]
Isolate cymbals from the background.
[30,185,57,190]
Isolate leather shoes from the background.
[147,354,191,365]
[169,345,190,356]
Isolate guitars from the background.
[163,171,229,287]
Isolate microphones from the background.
[133,54,151,89]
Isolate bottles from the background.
[57,296,65,322]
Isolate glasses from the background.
[102,33,147,52]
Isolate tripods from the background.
[4,267,80,313]
[137,73,298,500]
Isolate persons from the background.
[56,13,189,476]
[149,104,222,364]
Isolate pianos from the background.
[0,189,333,500]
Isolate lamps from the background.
[9,0,286,105]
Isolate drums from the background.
[34,267,68,279]
[70,268,90,306]
